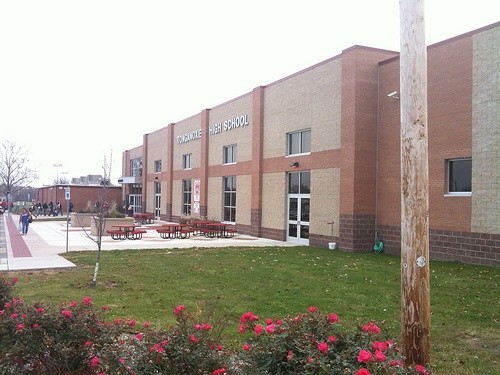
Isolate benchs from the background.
[106,229,147,241]
[157,228,238,239]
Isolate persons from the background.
[1,199,99,218]
[18,207,31,234]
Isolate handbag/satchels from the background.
[17,222,22,229]
[28,217,33,223]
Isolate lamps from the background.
[289,161,299,168]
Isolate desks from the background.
[163,224,189,238]
[133,213,155,224]
[112,225,141,240]
[194,220,232,237]
